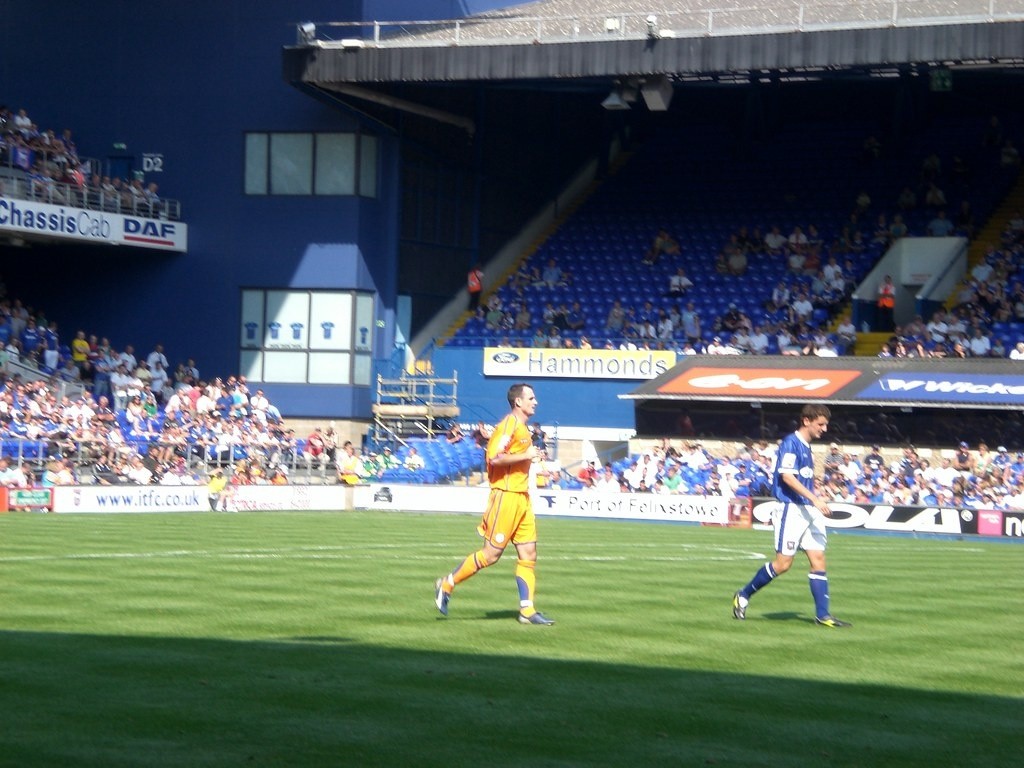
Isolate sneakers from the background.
[519,612,555,626]
[434,579,450,616]
[733,590,747,621]
[815,614,853,630]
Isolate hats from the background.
[1016,452,1023,458]
[997,446,1007,452]
[830,443,841,451]
[960,442,968,448]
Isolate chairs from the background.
[1,98,358,514]
[366,434,487,484]
[541,444,1023,513]
[456,198,990,358]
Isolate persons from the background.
[467,261,483,312]
[403,447,425,471]
[669,270,695,296]
[529,422,547,455]
[733,403,852,627]
[475,258,593,349]
[0,109,160,220]
[446,422,463,443]
[641,227,680,266]
[543,403,1024,510]
[435,384,556,625]
[707,336,726,354]
[923,155,968,176]
[470,421,488,449]
[984,117,1018,165]
[717,184,1024,359]
[865,139,882,162]
[602,298,708,355]
[0,299,401,511]
